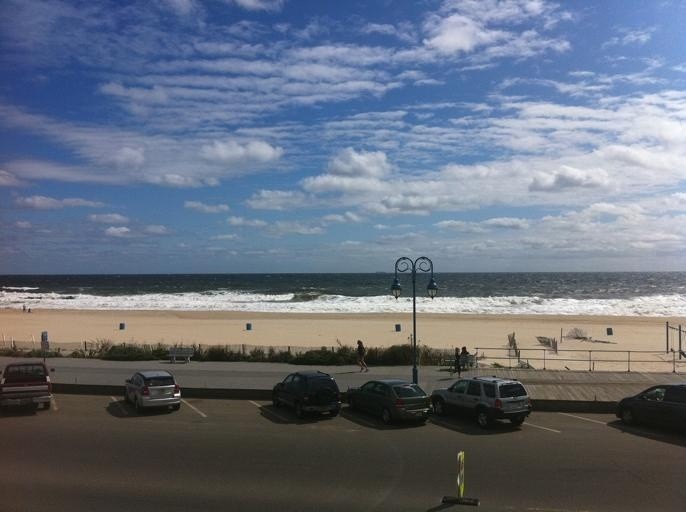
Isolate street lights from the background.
[392,254,440,385]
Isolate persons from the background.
[449,347,463,378]
[22,304,26,311]
[460,346,471,376]
[28,307,31,313]
[357,340,369,373]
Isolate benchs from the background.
[166,347,194,364]
[444,355,477,371]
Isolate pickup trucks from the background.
[0,360,56,413]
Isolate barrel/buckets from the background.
[120,322,124,330]
[247,323,251,330]
[607,328,613,335]
[396,324,401,332]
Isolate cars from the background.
[347,378,437,426]
[616,383,685,429]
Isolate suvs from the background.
[432,374,534,428]
[124,370,183,412]
[273,369,344,418]
